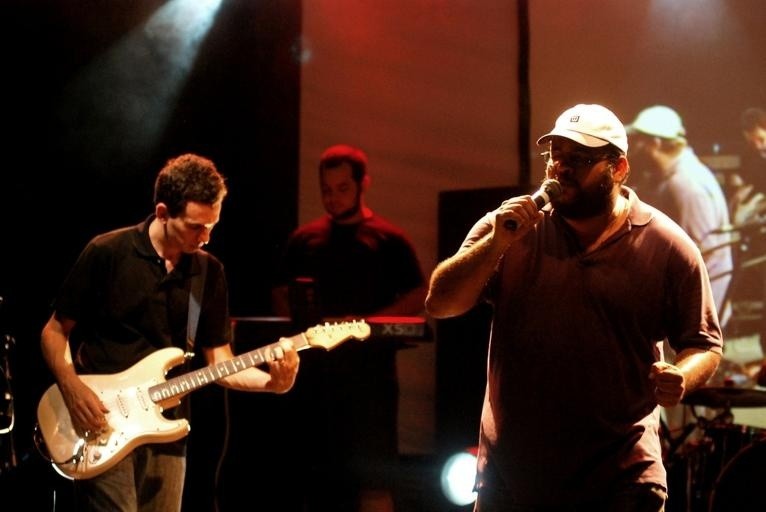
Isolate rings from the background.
[277,359,286,365]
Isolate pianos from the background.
[233,315,434,343]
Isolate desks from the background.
[182,317,427,512]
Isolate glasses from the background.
[540,151,607,176]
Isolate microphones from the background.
[502,178,562,233]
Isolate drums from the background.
[686,430,766,511]
[701,385,766,436]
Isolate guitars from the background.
[36,317,372,482]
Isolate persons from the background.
[623,104,735,453]
[424,103,724,511]
[270,141,430,511]
[723,107,765,359]
[38,152,301,511]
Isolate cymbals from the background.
[676,384,766,408]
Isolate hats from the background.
[537,103,628,154]
[624,104,686,139]
